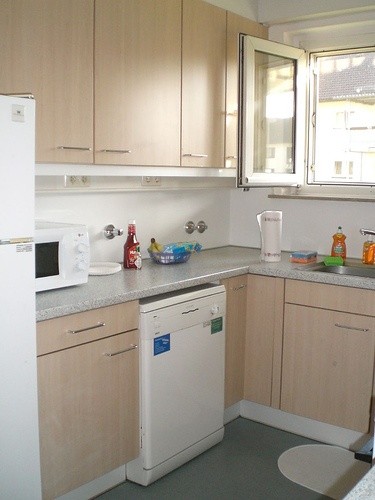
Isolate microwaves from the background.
[34,221,90,293]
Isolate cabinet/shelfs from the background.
[35,290,140,499]
[1,1,180,177]
[223,272,246,423]
[237,267,283,424]
[282,272,373,450]
[184,0,269,178]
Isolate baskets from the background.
[148,245,192,265]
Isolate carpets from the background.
[278,441,371,495]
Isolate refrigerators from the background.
[0,93,41,500]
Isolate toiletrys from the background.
[331,226,346,265]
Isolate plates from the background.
[90,262,122,276]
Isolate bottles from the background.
[331,225,347,260]
[124,220,142,270]
